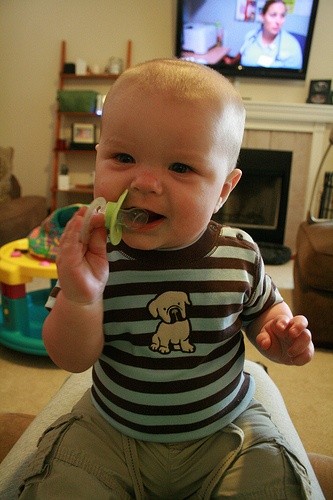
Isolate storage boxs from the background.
[60,89,98,113]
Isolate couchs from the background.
[0,147,47,250]
[294,219,333,348]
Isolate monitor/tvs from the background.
[177,0,318,80]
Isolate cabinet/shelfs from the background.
[50,40,132,214]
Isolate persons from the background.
[18,59,314,500]
[240,0,302,70]
